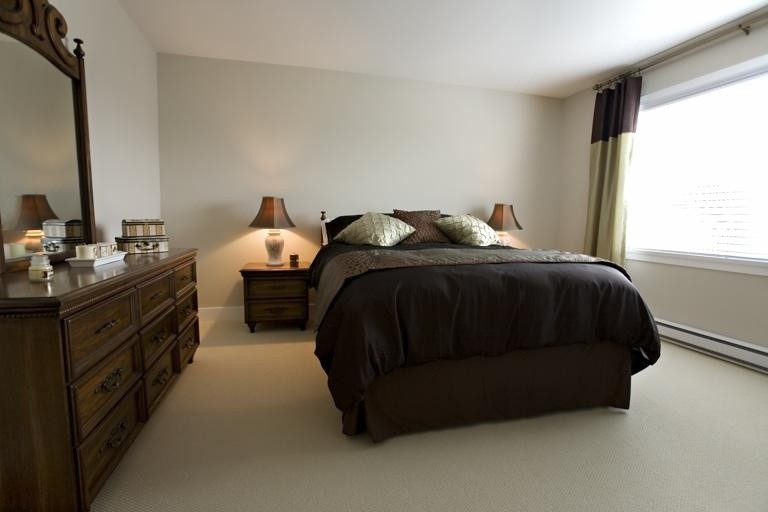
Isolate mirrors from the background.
[0,1,97,275]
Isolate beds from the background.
[315,210,660,444]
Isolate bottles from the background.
[27,256,54,282]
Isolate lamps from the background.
[248,195,297,267]
[486,203,524,244]
[17,194,59,252]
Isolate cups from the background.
[75,242,116,258]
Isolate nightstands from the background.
[238,262,316,333]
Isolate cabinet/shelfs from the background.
[1,245,201,512]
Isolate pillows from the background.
[333,207,502,248]
